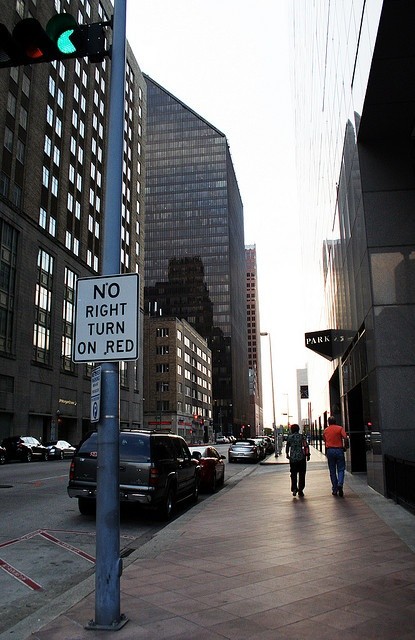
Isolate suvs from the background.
[228,436,275,463]
[67,428,201,522]
[1,435,48,462]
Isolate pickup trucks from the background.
[216,436,230,443]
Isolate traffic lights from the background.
[199,419,204,425]
[0,13,82,68]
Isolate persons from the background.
[286,424,310,496]
[322,416,349,497]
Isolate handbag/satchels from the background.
[300,434,309,456]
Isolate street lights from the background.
[260,332,277,457]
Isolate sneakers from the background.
[332,490,337,495]
[293,489,296,496]
[337,486,343,497]
[298,491,304,497]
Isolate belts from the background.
[329,447,342,449]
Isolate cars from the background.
[44,440,77,460]
[188,444,226,494]
